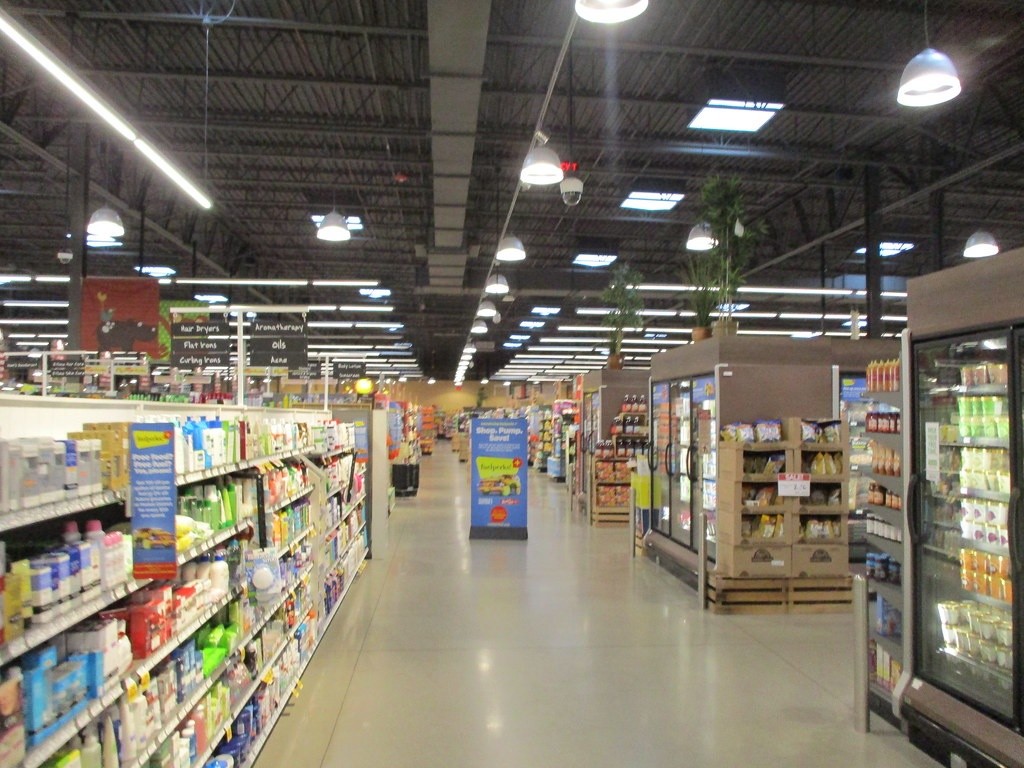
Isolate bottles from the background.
[865,552,900,584]
[170,412,304,475]
[866,513,902,542]
[865,412,900,434]
[868,482,901,510]
[62,521,127,590]
[871,447,901,477]
[156,552,230,592]
[595,394,649,459]
[866,358,900,392]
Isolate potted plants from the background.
[703,167,771,337]
[598,260,647,371]
[685,252,720,344]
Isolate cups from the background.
[937,363,1013,671]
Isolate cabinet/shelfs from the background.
[0,393,369,768]
[715,413,849,577]
[592,413,650,527]
[859,351,915,735]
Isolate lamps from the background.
[686,221,719,250]
[897,0,962,107]
[484,265,509,295]
[574,0,649,25]
[87,168,125,237]
[0,0,217,209]
[56,121,73,264]
[470,319,488,333]
[477,297,496,317]
[963,226,999,258]
[520,139,564,185]
[495,228,526,262]
[461,342,477,361]
[316,189,351,243]
